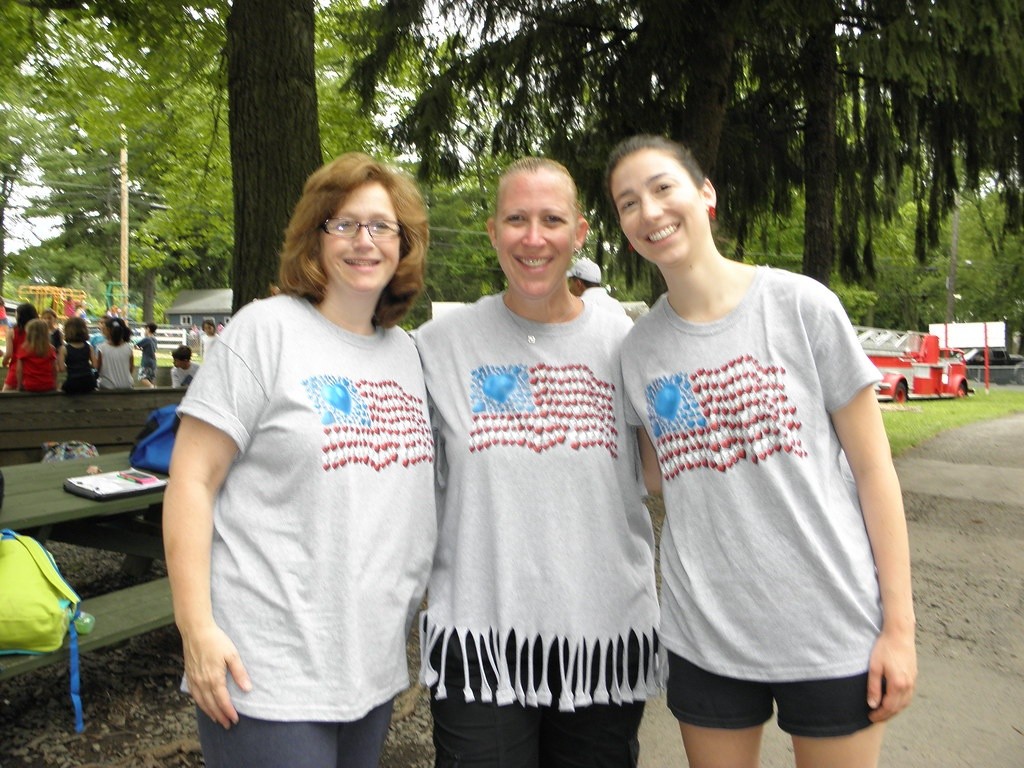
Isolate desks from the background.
[0,453,168,562]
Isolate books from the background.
[62,464,166,500]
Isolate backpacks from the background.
[0,529,78,654]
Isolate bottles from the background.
[72,610,95,635]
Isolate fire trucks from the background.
[853,326,968,403]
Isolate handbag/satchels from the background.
[129,405,179,472]
[38,439,97,464]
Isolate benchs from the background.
[0,386,188,470]
[0,575,175,685]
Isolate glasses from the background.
[323,216,403,237]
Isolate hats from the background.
[567,258,602,283]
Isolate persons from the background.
[0,296,227,394]
[405,157,668,768]
[606,137,916,768]
[568,254,631,323]
[162,149,428,768]
